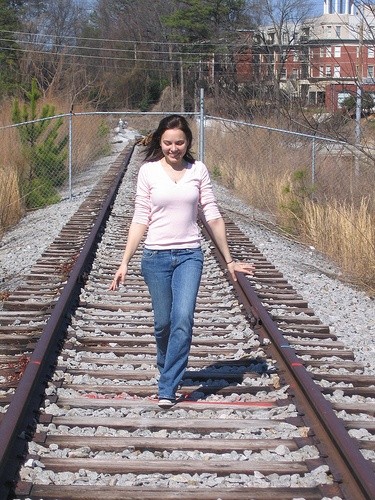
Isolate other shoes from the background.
[158,398,175,406]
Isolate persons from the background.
[109,114,256,406]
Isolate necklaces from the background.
[174,178,177,184]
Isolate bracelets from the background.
[227,260,233,264]
